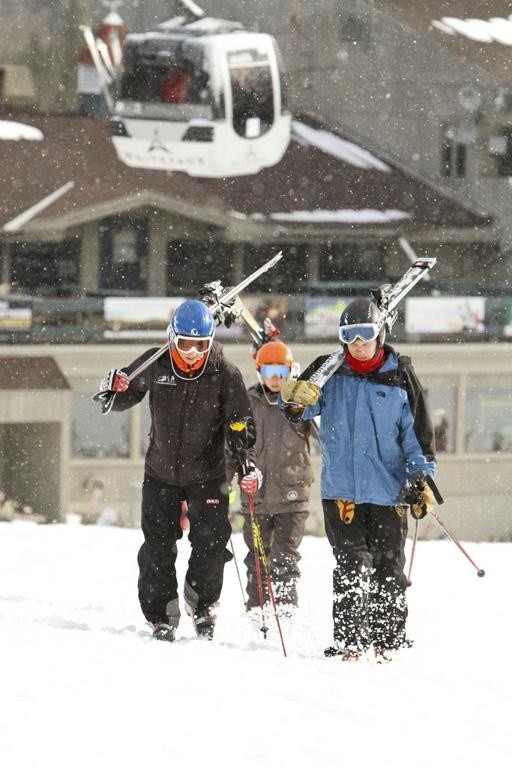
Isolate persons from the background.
[98,296,264,645]
[254,287,304,342]
[278,292,438,667]
[224,336,322,620]
[122,53,283,137]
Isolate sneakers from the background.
[188,604,219,641]
[148,622,176,642]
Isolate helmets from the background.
[339,299,386,348]
[166,297,216,354]
[256,340,293,371]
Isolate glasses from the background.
[261,364,291,380]
[173,333,213,354]
[339,322,379,344]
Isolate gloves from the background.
[281,381,322,406]
[98,368,130,393]
[239,461,264,495]
[411,487,436,519]
